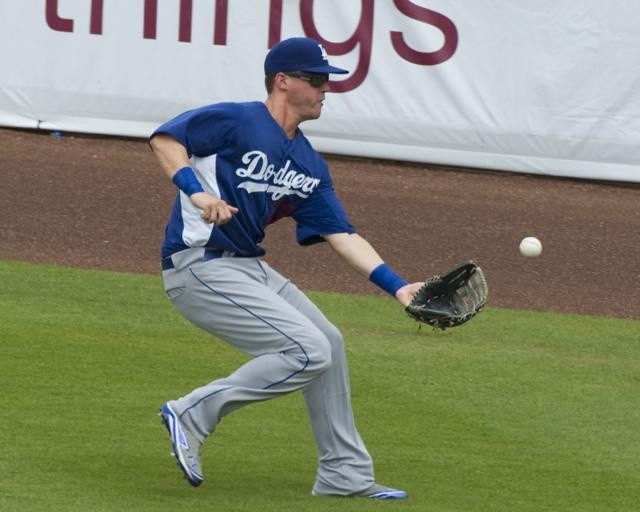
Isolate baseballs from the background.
[520,237,542,257]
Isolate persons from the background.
[145,36,428,500]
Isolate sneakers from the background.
[156,401,204,486]
[354,482,408,500]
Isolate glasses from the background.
[273,71,329,87]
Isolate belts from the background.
[161,249,225,270]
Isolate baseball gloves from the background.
[406,260,488,328]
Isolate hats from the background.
[265,37,349,74]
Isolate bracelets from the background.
[368,262,409,299]
[172,166,205,198]
